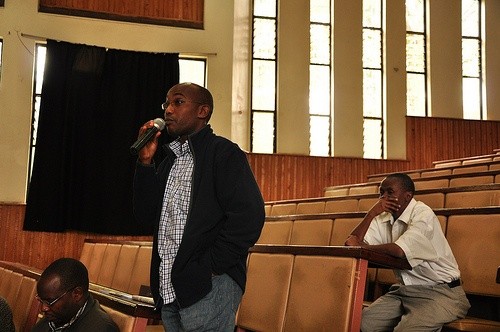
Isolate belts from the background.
[447,278,464,287]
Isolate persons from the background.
[31,258,120,332]
[345,173,471,332]
[132,83,265,331]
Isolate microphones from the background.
[130,118,166,153]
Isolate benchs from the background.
[1,148,500,330]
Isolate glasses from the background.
[35,286,76,307]
[161,99,204,110]
